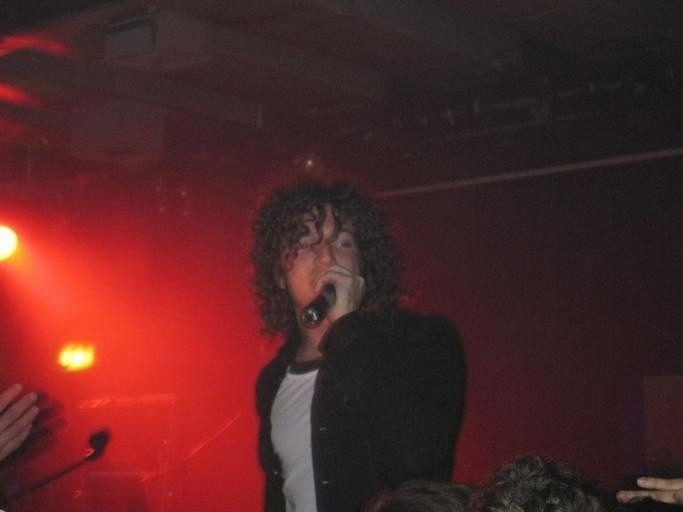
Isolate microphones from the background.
[299,284,338,329]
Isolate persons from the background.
[0,383,44,467]
[610,468,680,510]
[362,452,475,512]
[250,174,467,509]
[466,451,610,512]
